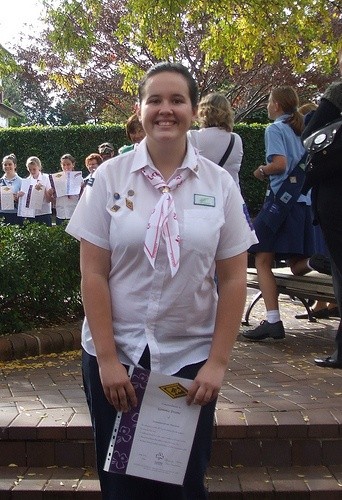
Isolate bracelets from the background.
[259,165,266,176]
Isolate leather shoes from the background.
[314,355,338,368]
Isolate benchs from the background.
[241,266,337,326]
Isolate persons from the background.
[245,70,342,368]
[64,62,259,500]
[188,94,244,291]
[0,113,150,227]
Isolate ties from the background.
[139,147,200,278]
[5,177,17,185]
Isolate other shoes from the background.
[295,306,329,319]
[309,254,332,276]
[242,319,285,340]
[328,306,339,317]
